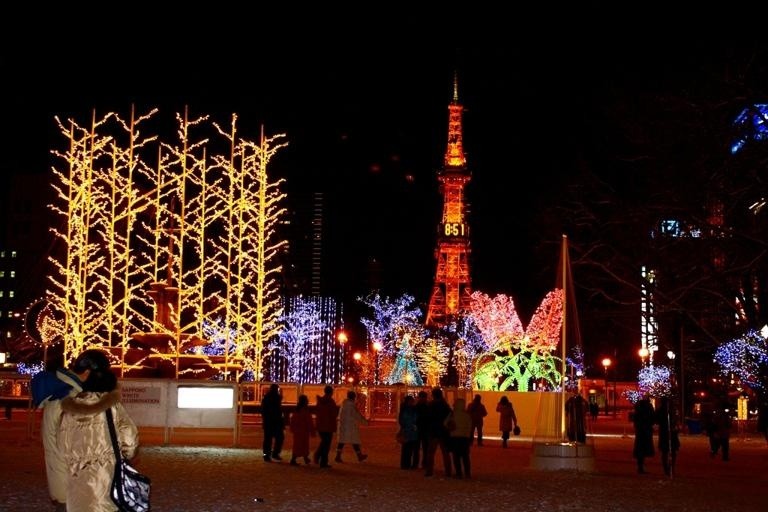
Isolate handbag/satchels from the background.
[110,459,151,512]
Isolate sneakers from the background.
[263,448,368,468]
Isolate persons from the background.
[314,385,341,468]
[701,400,731,463]
[564,386,600,448]
[260,384,285,462]
[496,396,517,447]
[60,368,139,511]
[288,395,316,465]
[30,349,110,511]
[467,394,486,446]
[628,398,655,475]
[398,389,472,480]
[335,391,371,464]
[655,396,681,478]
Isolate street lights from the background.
[638,349,648,400]
[353,351,362,385]
[372,341,381,385]
[338,333,347,385]
[602,358,611,416]
